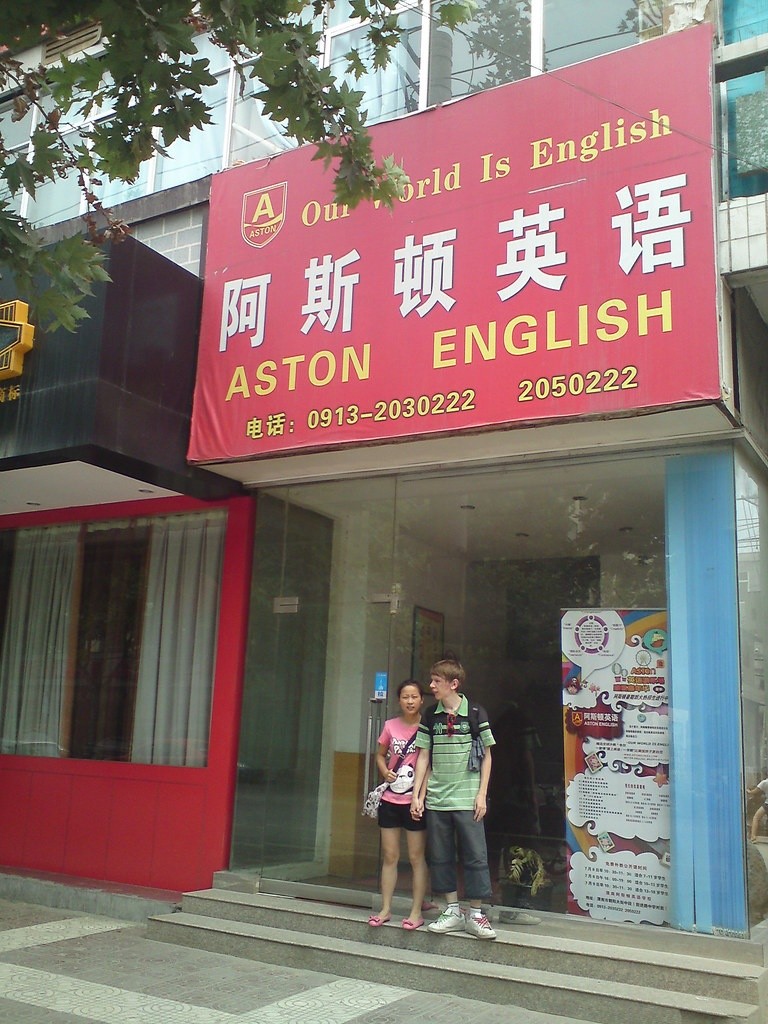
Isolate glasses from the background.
[447,713,456,736]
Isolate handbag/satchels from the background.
[361,781,391,819]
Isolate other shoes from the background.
[748,838,756,844]
[421,900,439,910]
[368,913,391,926]
[401,916,424,930]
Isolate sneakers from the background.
[465,912,496,939]
[427,912,467,933]
[498,910,540,925]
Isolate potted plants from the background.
[502,844,556,911]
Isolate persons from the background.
[367,678,430,929]
[484,694,543,910]
[410,660,498,941]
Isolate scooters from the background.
[746,785,768,836]
